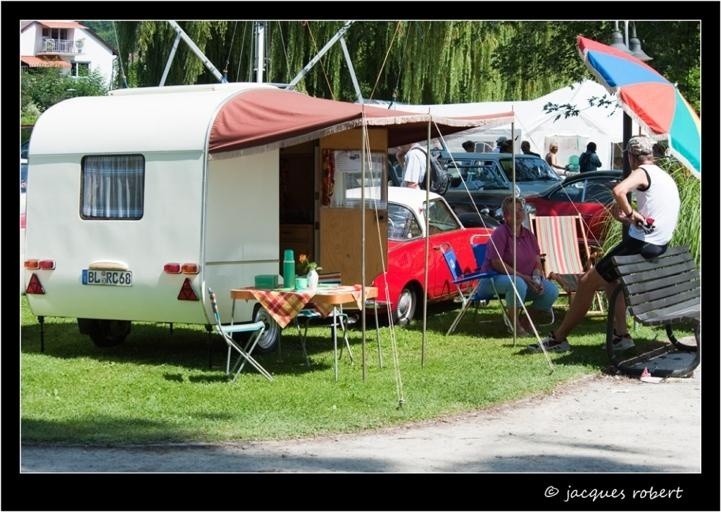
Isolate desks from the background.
[223,282,384,383]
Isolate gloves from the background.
[637,219,657,235]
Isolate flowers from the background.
[298,252,320,272]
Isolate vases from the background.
[306,268,319,289]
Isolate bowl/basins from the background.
[255,275,279,289]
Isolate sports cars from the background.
[342,185,498,327]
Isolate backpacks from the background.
[411,145,452,199]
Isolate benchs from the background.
[611,242,700,381]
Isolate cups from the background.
[533,273,541,290]
[296,278,308,290]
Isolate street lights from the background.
[605,21,655,255]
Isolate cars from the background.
[460,170,629,263]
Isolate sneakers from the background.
[527,330,572,354]
[600,327,637,352]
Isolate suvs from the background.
[390,149,579,231]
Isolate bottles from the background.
[284,249,296,288]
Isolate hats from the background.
[496,136,510,145]
[621,135,654,157]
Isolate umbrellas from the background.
[576,36,701,181]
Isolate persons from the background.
[461,137,540,180]
[396,141,435,191]
[477,197,559,337]
[546,142,566,171]
[580,142,602,173]
[527,136,680,353]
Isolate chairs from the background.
[527,212,604,317]
[297,270,354,369]
[435,240,517,337]
[469,232,556,327]
[207,285,276,387]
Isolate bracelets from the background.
[626,210,633,218]
